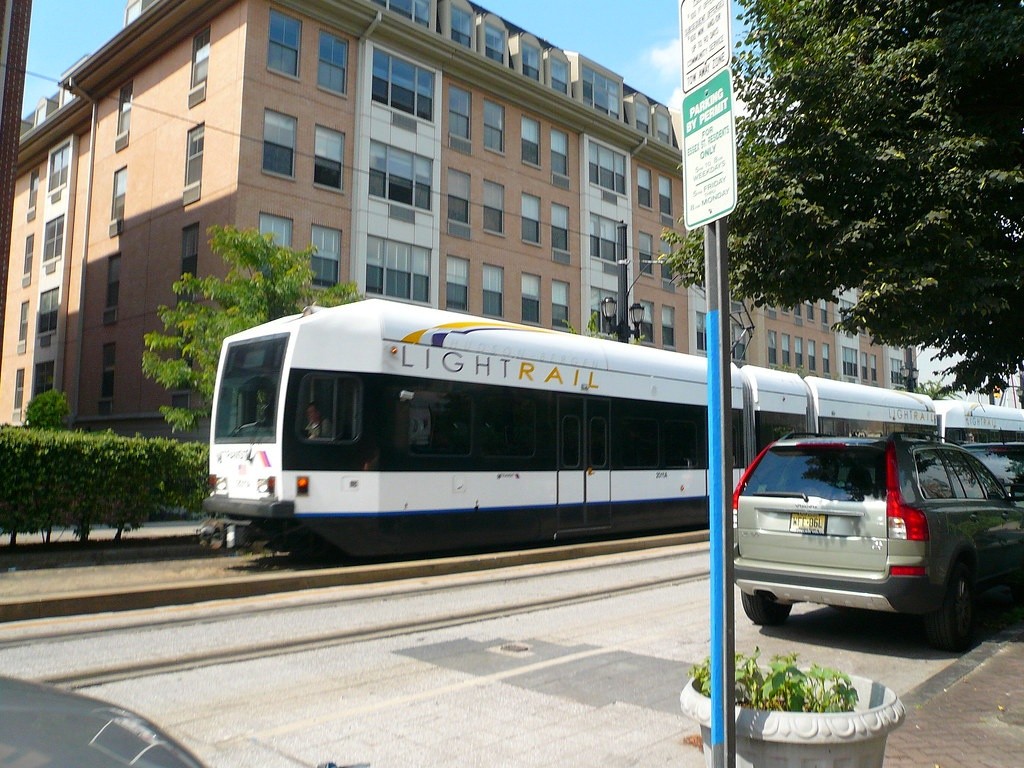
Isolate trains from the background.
[202,298,1024,566]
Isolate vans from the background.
[962,441,1024,510]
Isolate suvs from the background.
[732,430,1024,653]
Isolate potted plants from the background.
[681,647,906,768]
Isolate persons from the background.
[252,385,273,426]
[305,403,332,440]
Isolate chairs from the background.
[845,467,873,502]
